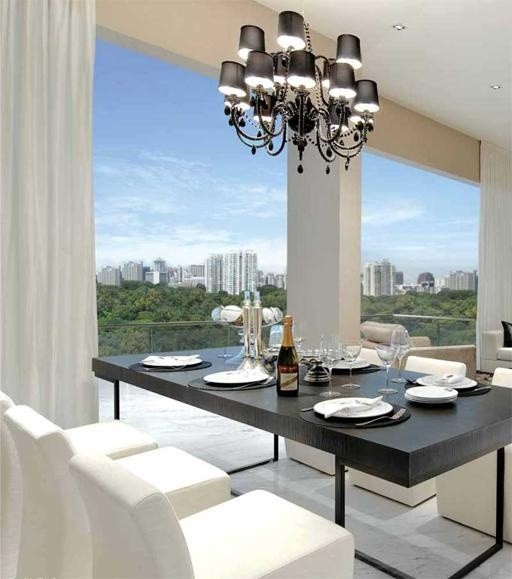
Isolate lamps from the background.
[218,1,380,174]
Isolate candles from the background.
[235,290,269,376]
